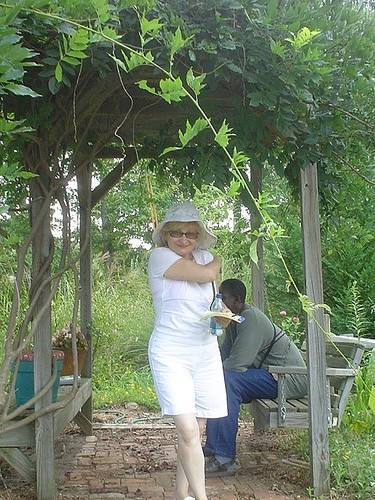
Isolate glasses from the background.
[165,230,200,240]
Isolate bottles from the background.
[209,294,225,337]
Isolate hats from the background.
[152,203,217,249]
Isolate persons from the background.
[201,279,309,477]
[145,202,236,500]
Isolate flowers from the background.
[52,322,86,350]
[15,348,65,361]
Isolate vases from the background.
[11,362,63,408]
[52,347,87,377]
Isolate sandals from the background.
[202,447,216,458]
[205,455,238,477]
